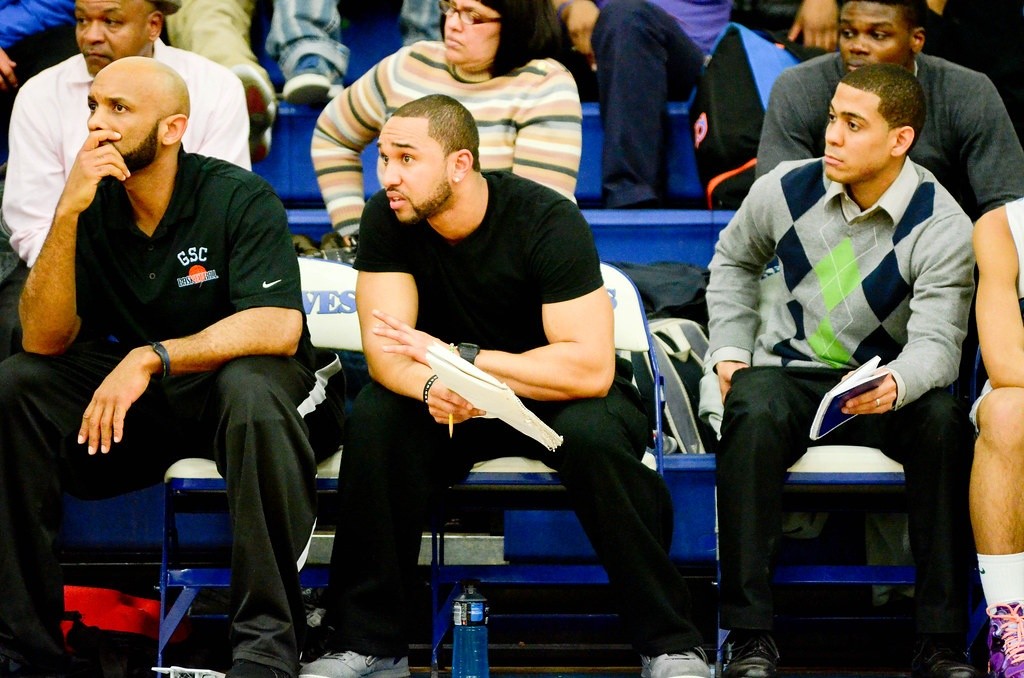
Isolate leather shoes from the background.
[909,635,977,678]
[722,631,784,678]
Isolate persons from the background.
[703,63,975,678]
[968,198,1024,678]
[0,55,345,678]
[0,0,1024,607]
[298,93,711,678]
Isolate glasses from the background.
[439,0,501,25]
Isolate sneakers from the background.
[295,649,412,678]
[641,651,712,678]
[224,658,289,678]
[985,600,1023,678]
[283,54,344,108]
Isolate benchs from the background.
[50,16,988,268]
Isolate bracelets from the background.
[423,374,438,405]
[556,0,573,23]
[150,341,171,383]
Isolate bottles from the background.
[320,233,343,266]
[452,586,489,678]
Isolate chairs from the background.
[156,255,920,678]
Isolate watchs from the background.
[457,342,480,365]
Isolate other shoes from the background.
[234,63,275,163]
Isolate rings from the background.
[876,398,880,406]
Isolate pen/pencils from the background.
[449,413,454,439]
